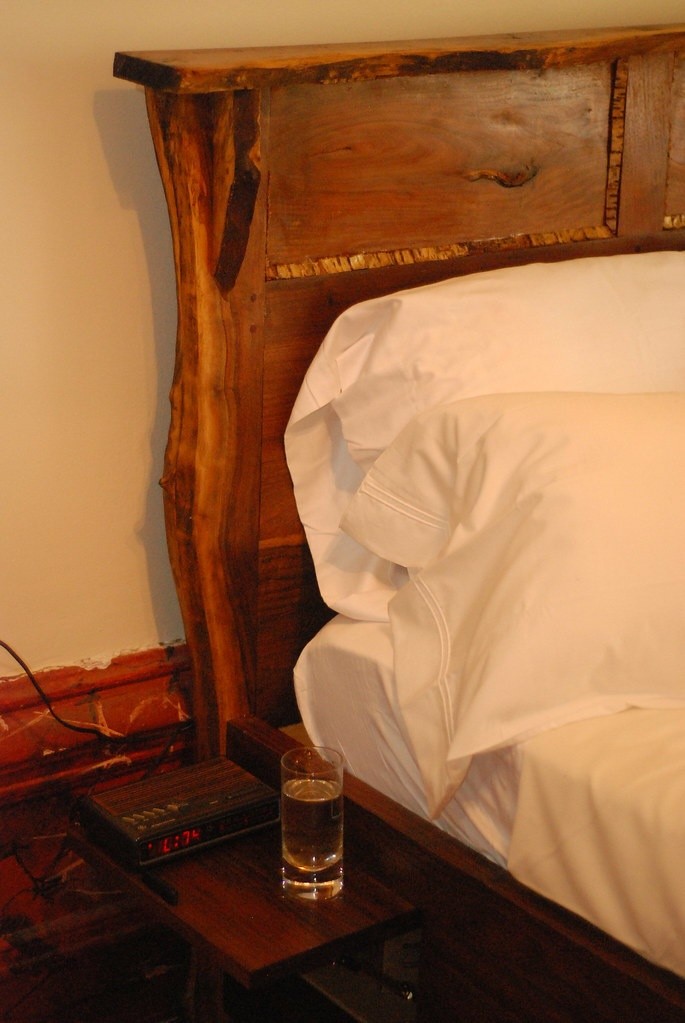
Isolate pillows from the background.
[338,391,685,822]
[284,252,685,619]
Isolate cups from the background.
[281,748,343,900]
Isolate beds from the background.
[112,24,684,1023]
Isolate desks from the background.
[0,758,417,1023]
